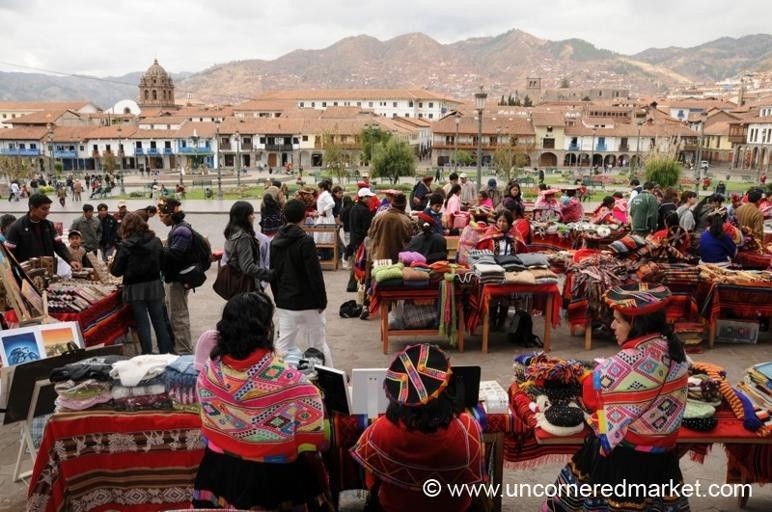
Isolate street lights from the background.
[215,117,223,197]
[589,127,597,178]
[473,84,487,198]
[297,132,302,184]
[116,126,126,194]
[454,112,460,174]
[387,128,399,177]
[634,120,642,180]
[579,135,584,168]
[49,127,56,188]
[236,127,241,187]
[363,123,380,180]
[695,111,708,200]
[495,126,509,167]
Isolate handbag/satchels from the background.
[212,263,248,300]
[177,262,209,292]
[507,310,544,350]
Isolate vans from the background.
[694,160,709,169]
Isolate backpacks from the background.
[173,223,214,271]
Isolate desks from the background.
[1,203,772,512]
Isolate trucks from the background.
[544,167,562,176]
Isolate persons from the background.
[0,161,772,368]
[347,343,498,511]
[188,291,338,512]
[543,280,691,512]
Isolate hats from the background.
[539,188,560,196]
[298,184,316,194]
[118,199,128,209]
[561,184,581,192]
[382,341,455,412]
[599,280,675,317]
[68,229,82,239]
[357,187,376,199]
